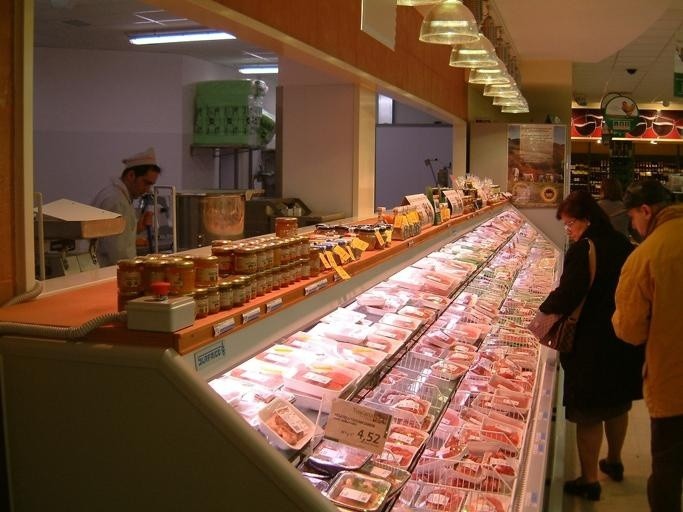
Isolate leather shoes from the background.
[596,456,625,482]
[561,476,601,501]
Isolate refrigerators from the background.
[1,195,577,512]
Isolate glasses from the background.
[561,216,577,233]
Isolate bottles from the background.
[573,158,666,192]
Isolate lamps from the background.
[238,64,278,74]
[127,30,238,46]
[396,0,530,114]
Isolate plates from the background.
[344,207,560,305]
[206,305,542,510]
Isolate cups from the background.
[201,195,245,244]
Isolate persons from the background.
[537,188,642,501]
[597,177,643,245]
[608,176,681,512]
[91,147,162,269]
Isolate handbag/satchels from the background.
[525,239,603,356]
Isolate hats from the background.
[619,177,668,209]
[120,146,162,172]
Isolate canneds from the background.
[116,216,394,320]
